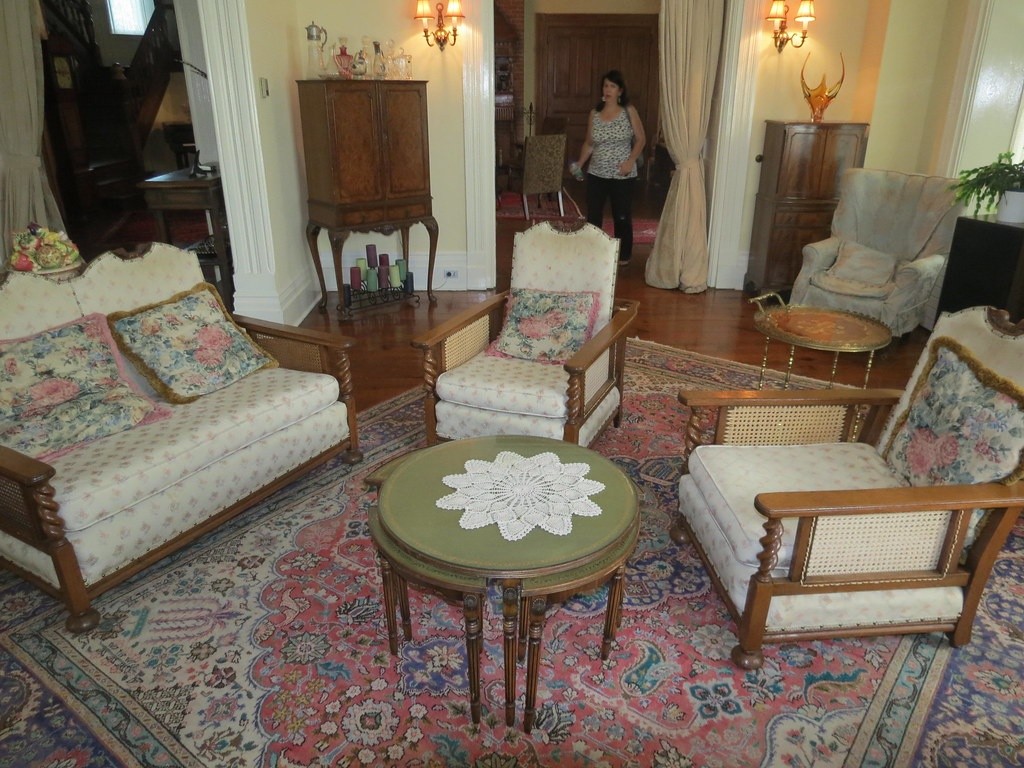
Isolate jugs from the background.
[306,21,327,79]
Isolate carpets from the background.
[0,339,1024,768]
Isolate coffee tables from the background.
[364,435,641,733]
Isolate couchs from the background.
[1,243,363,634]
[790,167,962,334]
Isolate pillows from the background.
[887,343,1024,491]
[108,286,276,401]
[1,311,165,464]
[489,290,598,365]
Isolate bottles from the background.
[337,37,353,79]
[373,41,388,80]
[571,162,584,182]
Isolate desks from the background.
[755,307,892,397]
[137,160,232,310]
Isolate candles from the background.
[344,242,414,306]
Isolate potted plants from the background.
[954,154,1024,223]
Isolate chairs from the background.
[498,135,566,219]
[667,307,1024,671]
[410,217,640,447]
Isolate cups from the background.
[393,48,412,79]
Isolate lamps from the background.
[412,0,466,52]
[765,1,815,53]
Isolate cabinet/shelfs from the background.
[296,78,439,319]
[937,218,1024,336]
[745,119,869,301]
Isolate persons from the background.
[569,69,646,266]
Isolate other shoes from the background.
[619,257,632,266]
